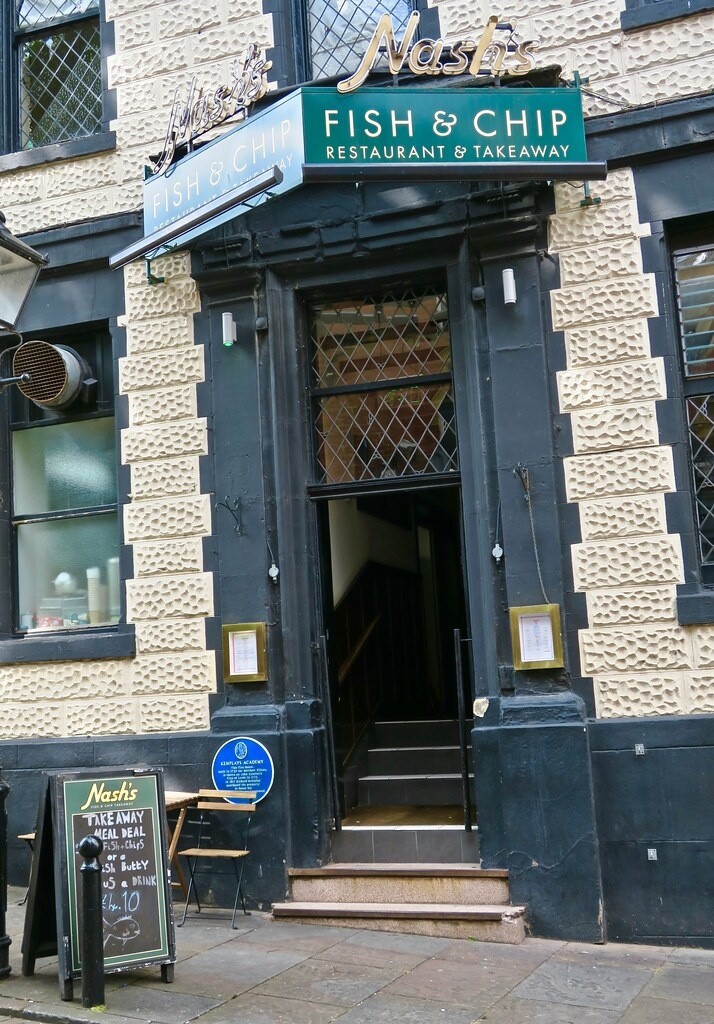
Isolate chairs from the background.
[177,789,257,930]
[17,833,36,906]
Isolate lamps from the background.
[221,311,238,348]
[503,267,518,305]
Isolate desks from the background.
[163,789,201,906]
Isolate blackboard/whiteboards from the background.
[21,766,178,981]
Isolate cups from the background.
[21,558,121,627]
